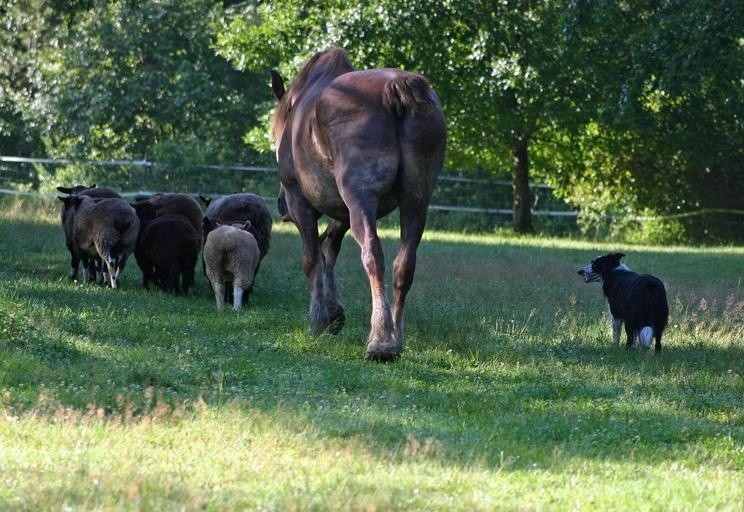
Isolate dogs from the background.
[574,250,669,354]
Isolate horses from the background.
[268,44,450,364]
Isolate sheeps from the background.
[53,180,273,314]
